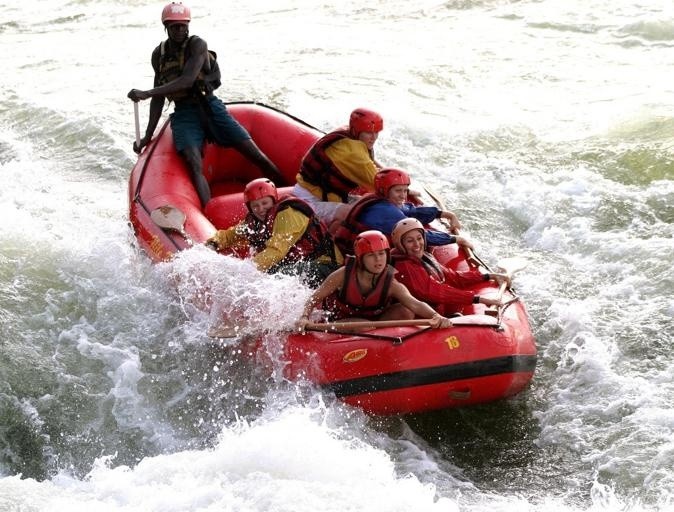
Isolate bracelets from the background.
[302,315,309,320]
[206,242,217,247]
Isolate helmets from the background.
[373,168,411,201]
[243,178,279,215]
[159,2,192,27]
[347,108,384,139]
[353,230,392,269]
[391,217,427,254]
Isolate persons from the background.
[389,218,512,317]
[295,230,453,333]
[206,178,344,290]
[127,3,287,208]
[293,107,421,235]
[329,168,473,259]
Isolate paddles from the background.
[207,317,440,339]
[485,257,527,314]
[150,205,198,248]
[421,185,485,266]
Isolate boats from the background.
[126,101,537,419]
